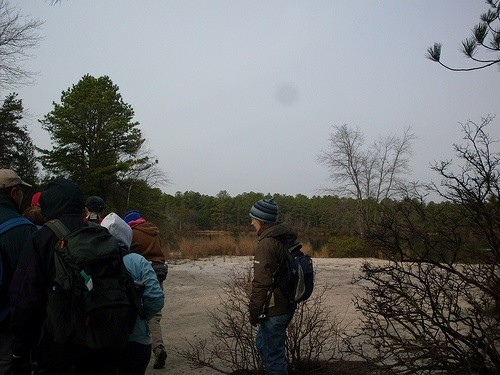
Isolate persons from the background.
[247,195,315,375]
[0,168,169,375]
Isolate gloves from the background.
[12,354,26,375]
[249,311,261,327]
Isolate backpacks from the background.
[271,235,313,304]
[43,218,139,343]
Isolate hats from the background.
[123,209,141,223]
[249,198,279,223]
[31,191,42,206]
[0,168,32,189]
[87,196,104,212]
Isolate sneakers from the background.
[153,345,167,369]
